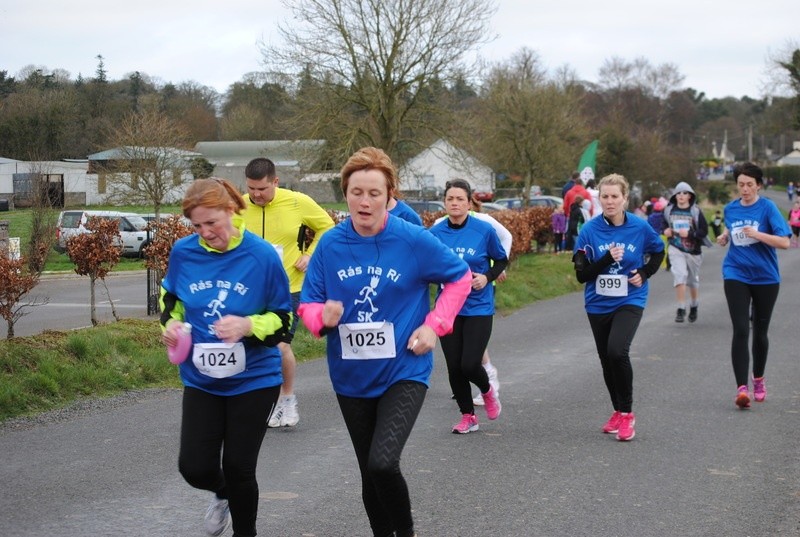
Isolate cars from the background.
[137,211,194,234]
[402,193,569,221]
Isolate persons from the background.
[572,173,667,442]
[660,181,708,323]
[633,196,671,272]
[297,147,473,537]
[387,178,512,434]
[552,172,602,255]
[710,210,724,238]
[787,181,800,248]
[158,177,294,537]
[717,162,793,410]
[233,158,335,428]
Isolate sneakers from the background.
[205,496,232,536]
[674,308,686,322]
[616,412,636,440]
[735,385,752,409]
[451,414,480,436]
[605,412,620,433]
[482,385,502,420]
[282,395,298,426]
[268,399,282,426]
[688,305,698,321]
[752,374,764,401]
[472,381,499,406]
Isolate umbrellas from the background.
[700,161,718,168]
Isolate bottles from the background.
[168,321,192,365]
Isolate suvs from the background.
[53,209,157,260]
